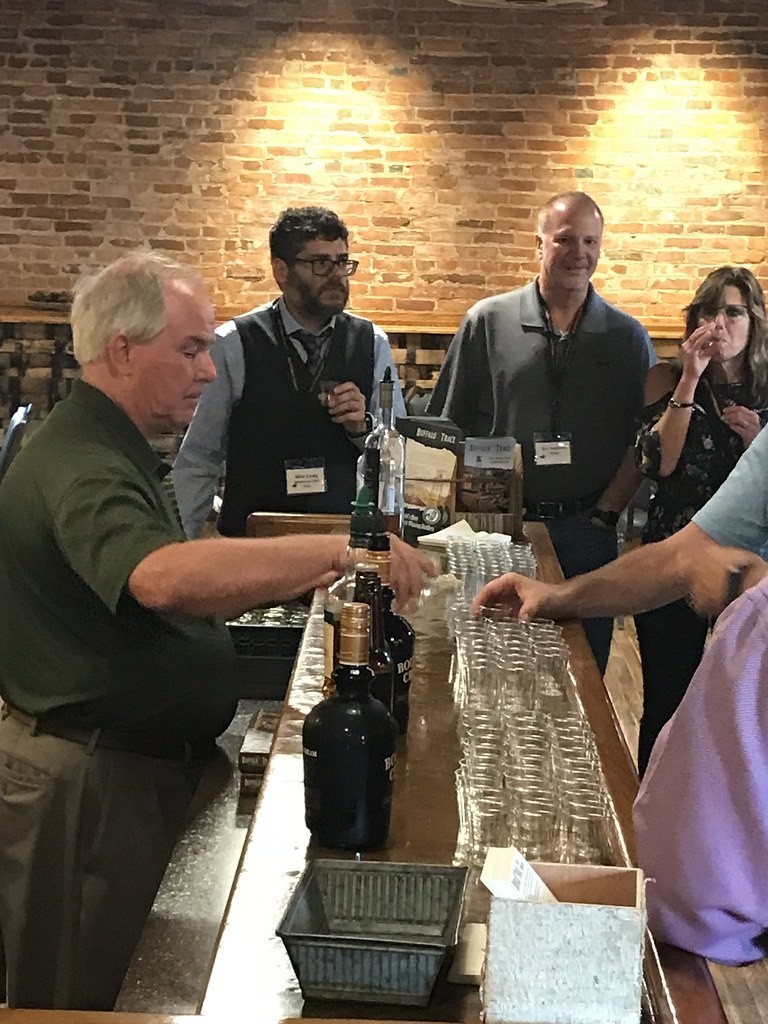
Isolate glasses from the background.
[278,257,358,277]
[697,305,749,319]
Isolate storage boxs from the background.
[483,860,646,1023]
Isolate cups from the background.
[452,604,621,864]
[319,378,341,407]
[446,537,536,592]
[702,329,725,345]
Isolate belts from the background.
[0,699,217,761]
[459,491,597,518]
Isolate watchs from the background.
[346,411,374,438]
[590,508,618,526]
[669,398,695,409]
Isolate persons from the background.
[424,192,658,680]
[636,263,768,782]
[0,249,439,1011]
[471,420,768,622]
[171,202,405,538]
[632,580,768,967]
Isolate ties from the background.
[291,327,332,395]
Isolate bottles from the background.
[347,380,404,560]
[300,561,418,854]
